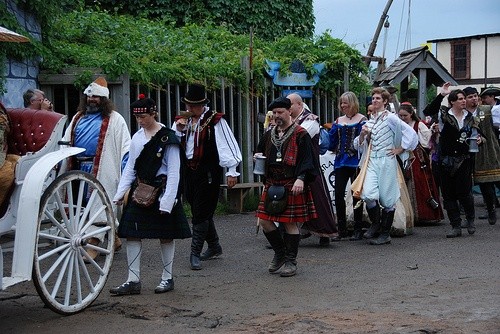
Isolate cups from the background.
[253,155,266,175]
[465,138,479,153]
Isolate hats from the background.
[131,94,157,115]
[479,87,500,97]
[83,77,109,99]
[181,84,210,105]
[267,97,291,111]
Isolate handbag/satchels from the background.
[264,186,288,214]
[132,184,155,207]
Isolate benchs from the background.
[2,105,68,184]
[220,183,263,214]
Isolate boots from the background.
[200,220,222,259]
[262,228,287,272]
[82,237,99,262]
[279,233,300,276]
[350,221,363,240]
[190,219,209,269]
[369,208,396,245]
[100,233,121,255]
[331,223,347,241]
[364,205,380,238]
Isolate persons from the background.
[110,94,191,295]
[283,82,500,246]
[251,97,336,277]
[23,89,53,112]
[61,76,132,263]
[171,83,243,271]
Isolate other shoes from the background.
[467,219,475,234]
[155,278,174,293]
[446,225,462,237]
[488,208,496,225]
[110,281,141,294]
[461,221,467,227]
[479,209,488,218]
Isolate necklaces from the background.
[270,126,296,147]
[346,114,355,120]
[293,108,303,122]
